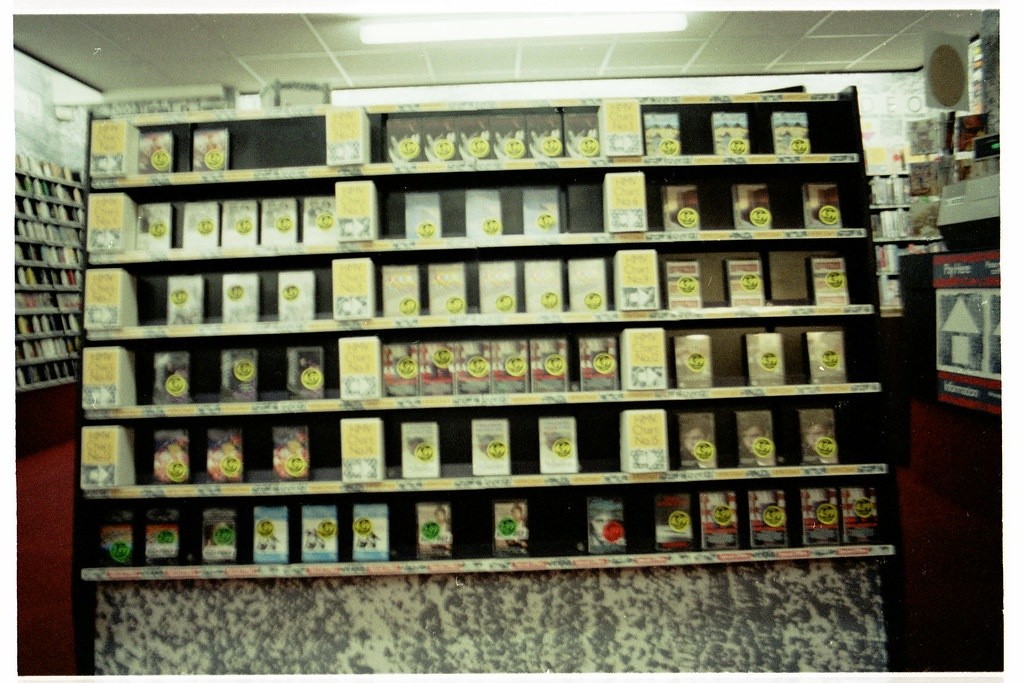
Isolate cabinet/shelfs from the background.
[17,65,1004,672]
